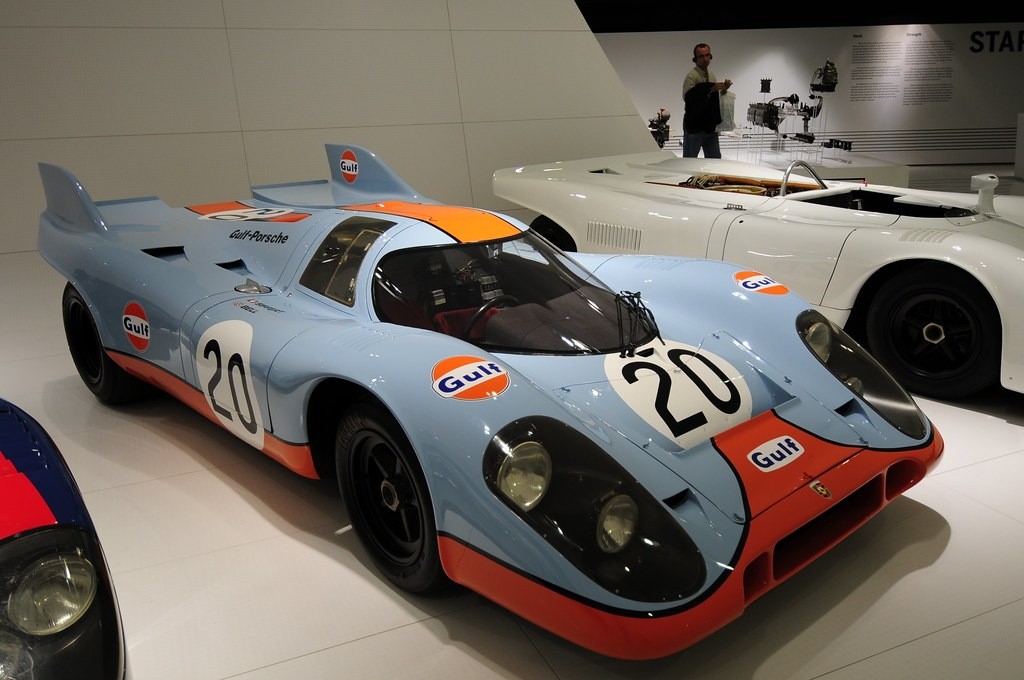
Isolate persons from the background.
[682,42,734,160]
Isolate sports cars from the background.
[0,398,126,680]
[493,151,1024,404]
[34,144,943,664]
